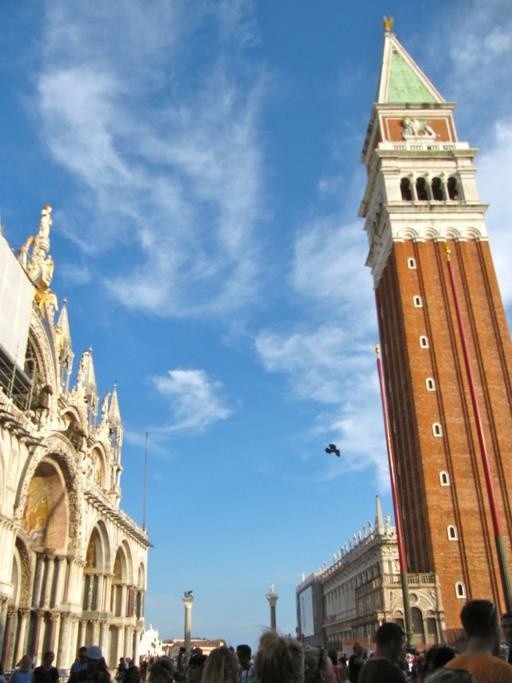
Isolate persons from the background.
[256,599,511,682]
[10,651,60,683]
[116,645,254,682]
[68,645,112,682]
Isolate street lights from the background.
[182,595,193,651]
[266,585,278,632]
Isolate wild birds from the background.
[325,444,340,456]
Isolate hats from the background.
[85,645,103,660]
